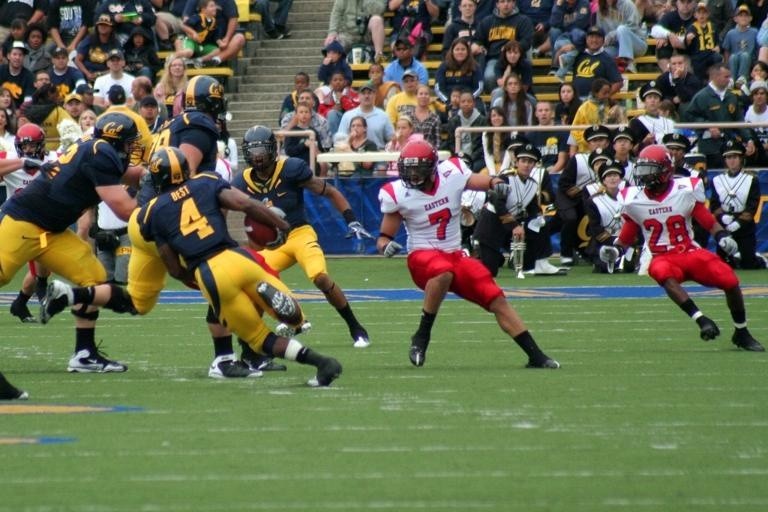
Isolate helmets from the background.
[147,145,192,193]
[241,124,278,171]
[397,138,439,191]
[633,143,676,192]
[93,111,139,147]
[183,74,226,115]
[14,122,46,157]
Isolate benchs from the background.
[157,0,262,93]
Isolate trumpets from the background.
[510,222,526,281]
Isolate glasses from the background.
[395,46,409,52]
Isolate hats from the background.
[105,48,125,64]
[639,78,665,102]
[505,134,542,162]
[63,82,100,104]
[96,13,113,26]
[583,124,747,182]
[401,69,417,80]
[586,25,606,37]
[3,40,30,57]
[358,82,378,93]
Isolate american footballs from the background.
[244,216,282,245]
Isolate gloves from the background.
[599,243,624,264]
[726,220,741,233]
[381,239,403,258]
[714,229,739,257]
[722,215,734,226]
[344,220,377,241]
[490,180,512,200]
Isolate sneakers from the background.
[275,27,292,37]
[408,332,431,368]
[535,261,558,274]
[263,29,284,40]
[525,353,560,369]
[696,315,720,341]
[731,327,766,352]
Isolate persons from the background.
[598,142,766,354]
[0,74,372,402]
[0,1,766,277]
[376,138,562,370]
